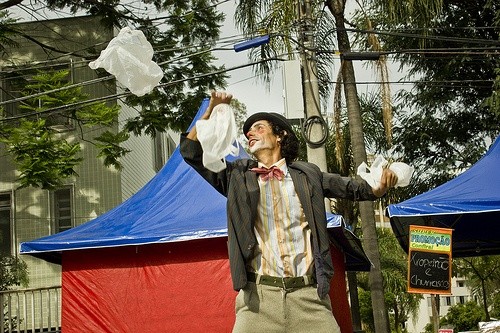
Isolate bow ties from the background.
[250,166,285,181]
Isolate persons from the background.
[179,90,398,333]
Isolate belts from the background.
[247,272,316,287]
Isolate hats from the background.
[243,112,300,145]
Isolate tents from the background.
[16,94,380,333]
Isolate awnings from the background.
[385,131,500,255]
